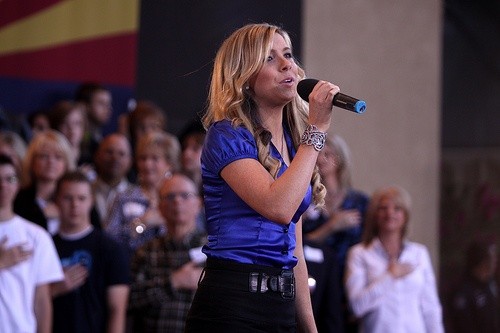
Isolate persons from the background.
[344,186,444,333]
[301,136,372,333]
[0,81,207,333]
[186,23,340,333]
[439,240,500,333]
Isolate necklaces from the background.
[280,133,284,154]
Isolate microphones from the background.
[297,79,366,114]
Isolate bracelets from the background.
[301,123,327,151]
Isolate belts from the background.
[206,270,296,301]
